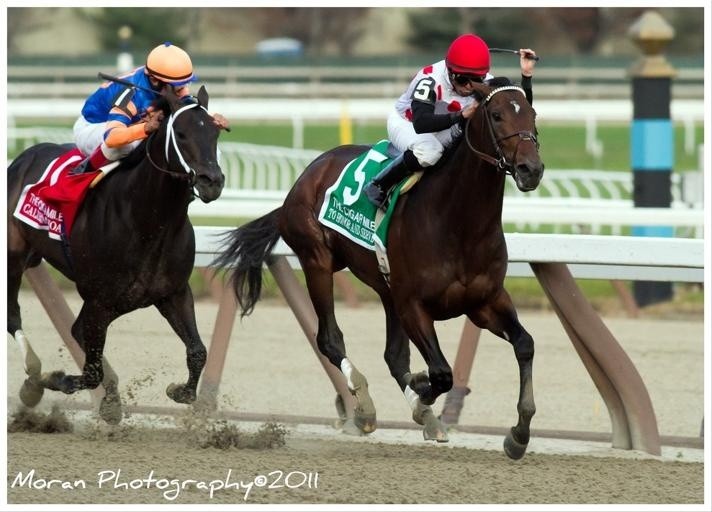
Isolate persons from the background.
[69,43,231,185]
[357,27,541,213]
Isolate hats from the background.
[444,33,491,75]
[142,42,198,86]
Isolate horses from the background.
[202,76,545,461]
[7,84,225,427]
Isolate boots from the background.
[363,149,424,211]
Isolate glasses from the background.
[449,69,487,87]
